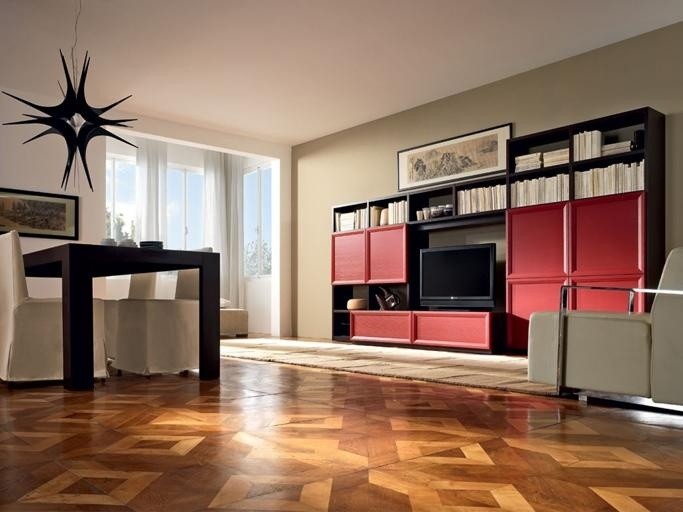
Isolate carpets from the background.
[220,337,560,394]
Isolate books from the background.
[335,200,407,232]
[573,131,633,163]
[574,158,644,199]
[457,183,507,215]
[511,173,570,209]
[514,147,569,172]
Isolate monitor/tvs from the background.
[420,243,495,308]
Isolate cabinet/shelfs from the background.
[506,104,664,356]
[331,172,508,353]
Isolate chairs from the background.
[0,227,221,393]
[525,242,682,407]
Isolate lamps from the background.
[2,49,139,190]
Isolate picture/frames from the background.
[0,185,81,241]
[396,124,513,191]
